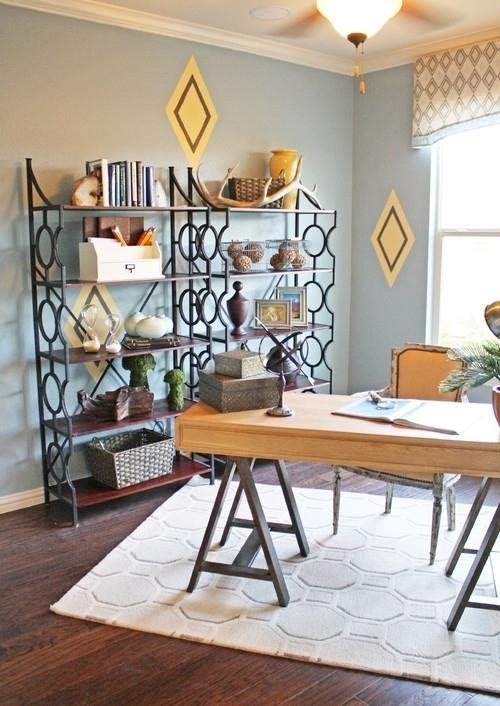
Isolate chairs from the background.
[333,343,469,565]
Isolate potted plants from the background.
[438,339,500,426]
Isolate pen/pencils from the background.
[137,225,157,246]
[111,225,128,246]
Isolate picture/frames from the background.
[253,300,292,330]
[275,287,308,327]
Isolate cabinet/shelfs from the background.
[26,158,215,528]
[188,167,337,465]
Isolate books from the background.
[331,397,479,435]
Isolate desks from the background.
[175,393,500,631]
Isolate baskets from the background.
[86,421,176,489]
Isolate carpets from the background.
[50,474,500,694]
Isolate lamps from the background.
[315,1,402,95]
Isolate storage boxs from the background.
[199,370,285,412]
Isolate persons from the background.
[86,158,155,207]
